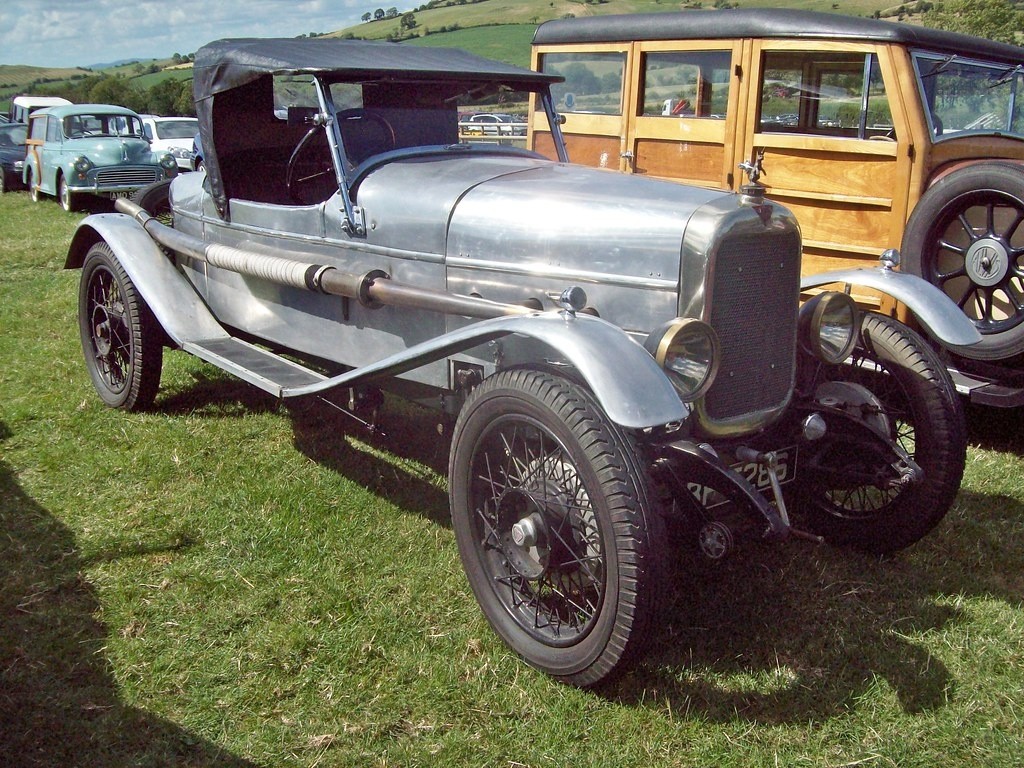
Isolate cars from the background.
[22,103,180,212]
[526,18,1024,409]
[458,110,527,136]
[60,36,984,688]
[189,132,206,171]
[0,123,29,193]
[120,115,201,173]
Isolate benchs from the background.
[218,147,375,206]
